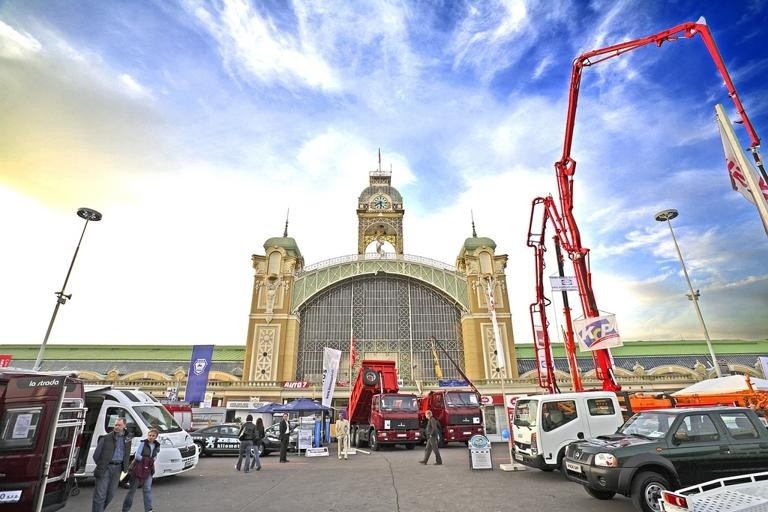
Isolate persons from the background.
[418,410,442,465]
[335,413,348,460]
[279,413,290,463]
[92,417,131,512]
[234,414,265,473]
[122,429,160,512]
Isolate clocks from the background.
[369,193,390,214]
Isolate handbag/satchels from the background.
[238,426,247,440]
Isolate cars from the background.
[187,424,285,457]
[262,421,321,453]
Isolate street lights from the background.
[32,204,104,374]
[652,205,722,377]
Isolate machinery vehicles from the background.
[509,23,768,474]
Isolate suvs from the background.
[561,404,767,509]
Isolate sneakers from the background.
[280,459,290,463]
[256,466,261,471]
[235,465,240,471]
[433,462,442,465]
[244,471,251,473]
[418,461,427,464]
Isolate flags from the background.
[484,282,507,379]
[349,328,356,370]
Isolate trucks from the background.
[0,362,198,511]
[342,353,486,450]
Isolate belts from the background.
[109,462,123,465]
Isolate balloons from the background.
[501,428,510,439]
